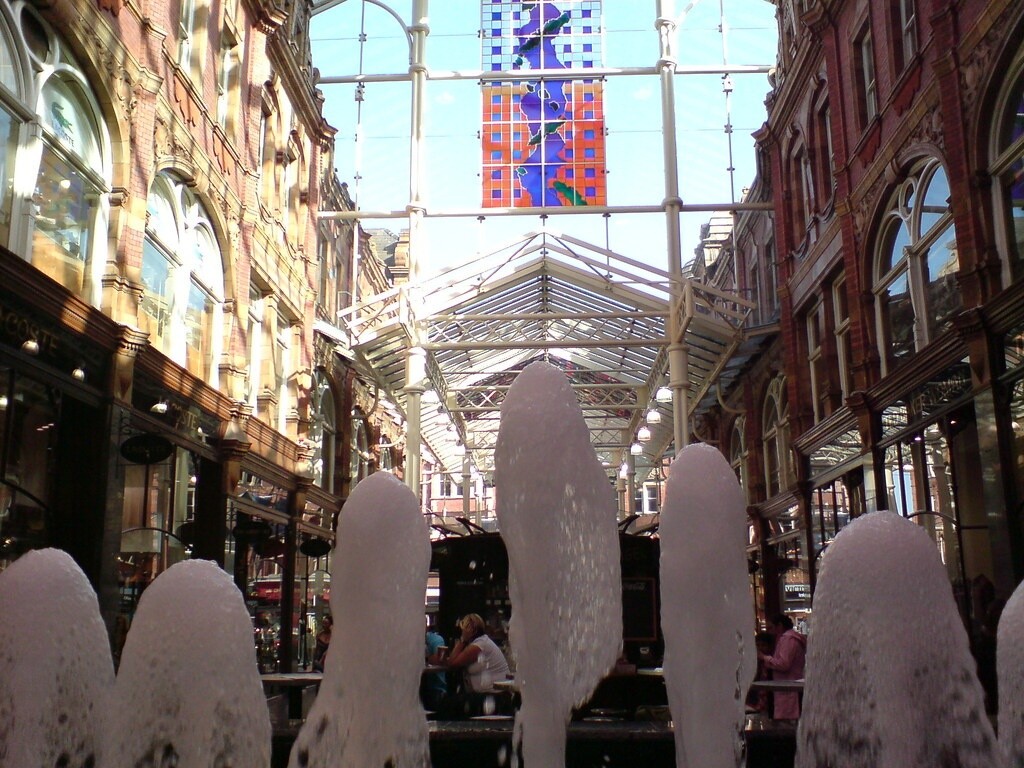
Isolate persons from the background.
[441,612,510,721]
[420,617,447,709]
[312,616,333,696]
[754,632,775,652]
[757,614,808,720]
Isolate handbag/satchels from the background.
[446,669,466,704]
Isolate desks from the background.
[260,666,805,720]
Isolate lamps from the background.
[638,426,650,441]
[656,387,673,403]
[647,409,661,423]
[117,409,172,465]
[630,444,643,455]
